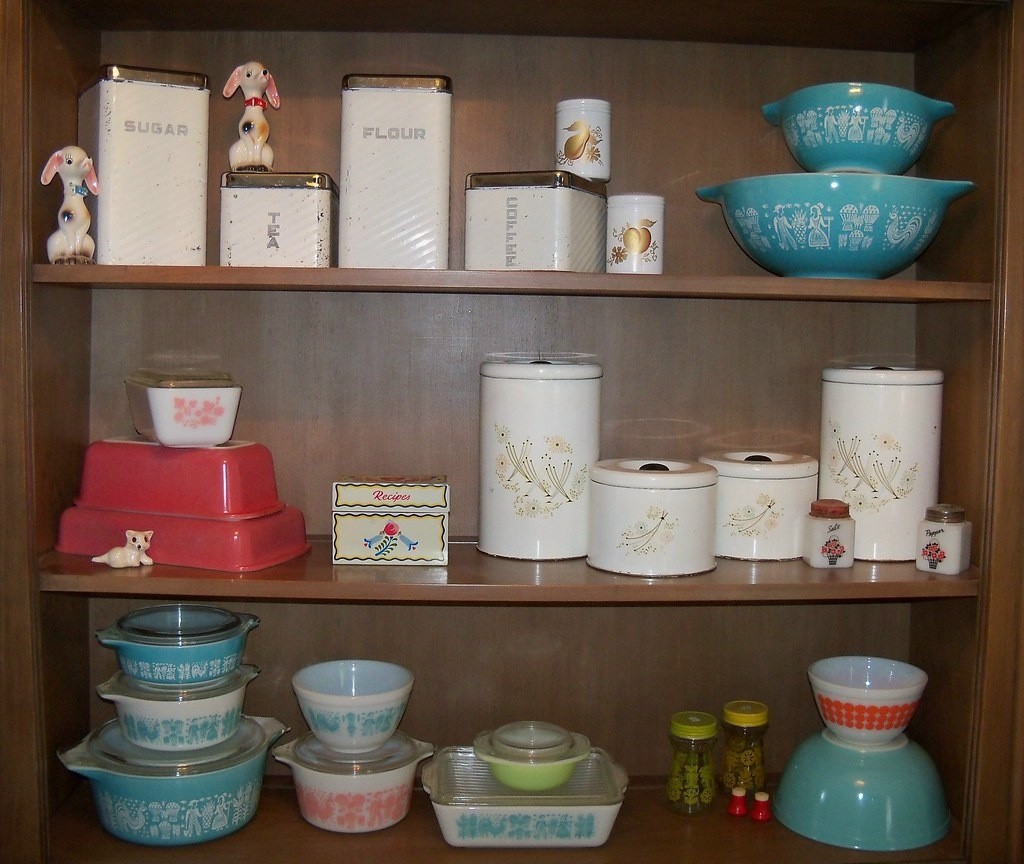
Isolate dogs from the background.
[91,530,153,569]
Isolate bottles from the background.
[819,364,946,562]
[717,700,770,800]
[802,497,858,570]
[699,448,820,561]
[475,361,608,559]
[665,711,719,818]
[914,503,973,577]
[585,459,717,579]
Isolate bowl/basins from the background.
[473,720,591,791]
[694,82,976,280]
[421,745,629,849]
[807,655,929,746]
[57,604,290,848]
[273,659,437,833]
[772,729,952,853]
[124,366,241,444]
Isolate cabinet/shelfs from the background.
[0,0,1024,864]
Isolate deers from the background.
[223,62,280,173]
[40,146,99,263]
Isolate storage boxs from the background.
[124,367,243,448]
[330,474,451,564]
[419,746,630,848]
[78,62,608,275]
